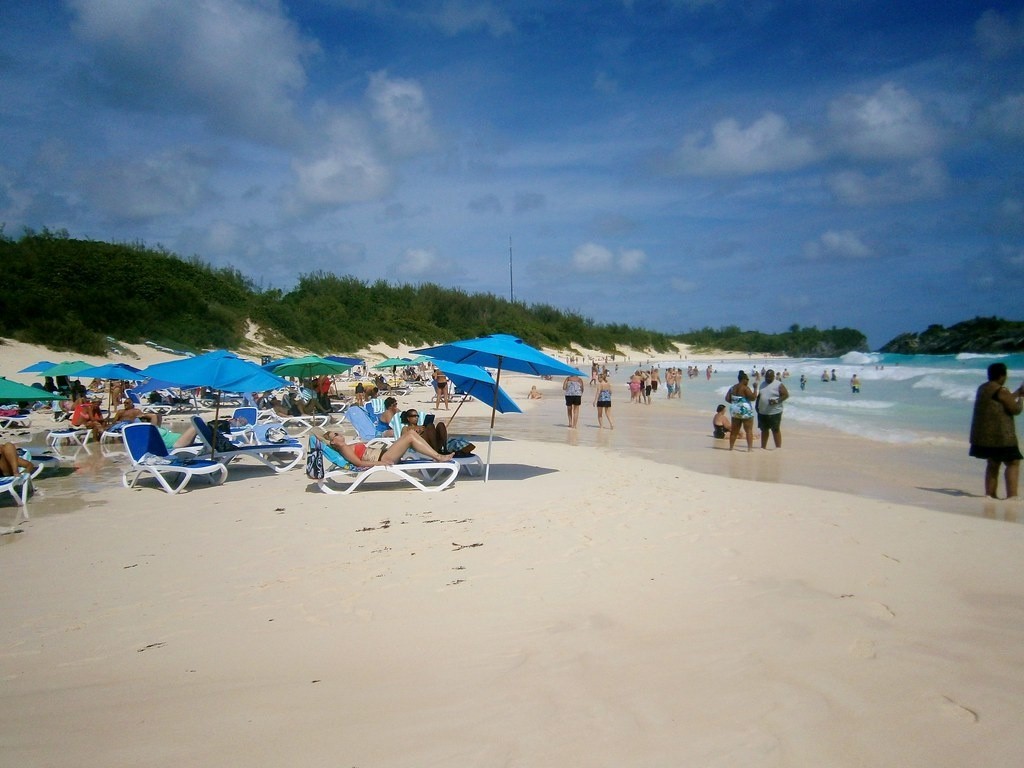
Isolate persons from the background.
[356,383,378,406]
[323,397,454,464]
[706,363,861,452]
[630,366,661,404]
[527,386,543,399]
[434,370,449,410]
[688,365,699,378]
[665,368,682,398]
[590,363,613,428]
[268,375,338,423]
[144,414,198,447]
[44,376,144,441]
[969,363,1024,499]
[0,442,34,477]
[563,368,583,427]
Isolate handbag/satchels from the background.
[306,449,324,479]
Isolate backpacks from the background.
[214,430,235,450]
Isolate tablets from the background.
[423,415,435,428]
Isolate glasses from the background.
[124,402,130,404]
[746,378,749,380]
[598,376,602,378]
[408,414,419,417]
[332,433,338,441]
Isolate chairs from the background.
[189,415,304,474]
[0,387,348,447]
[0,452,61,506]
[344,375,486,477]
[121,423,229,495]
[307,434,460,495]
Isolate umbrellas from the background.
[137,350,293,464]
[372,355,429,386]
[273,356,353,427]
[410,334,588,482]
[0,361,148,423]
[324,356,364,397]
